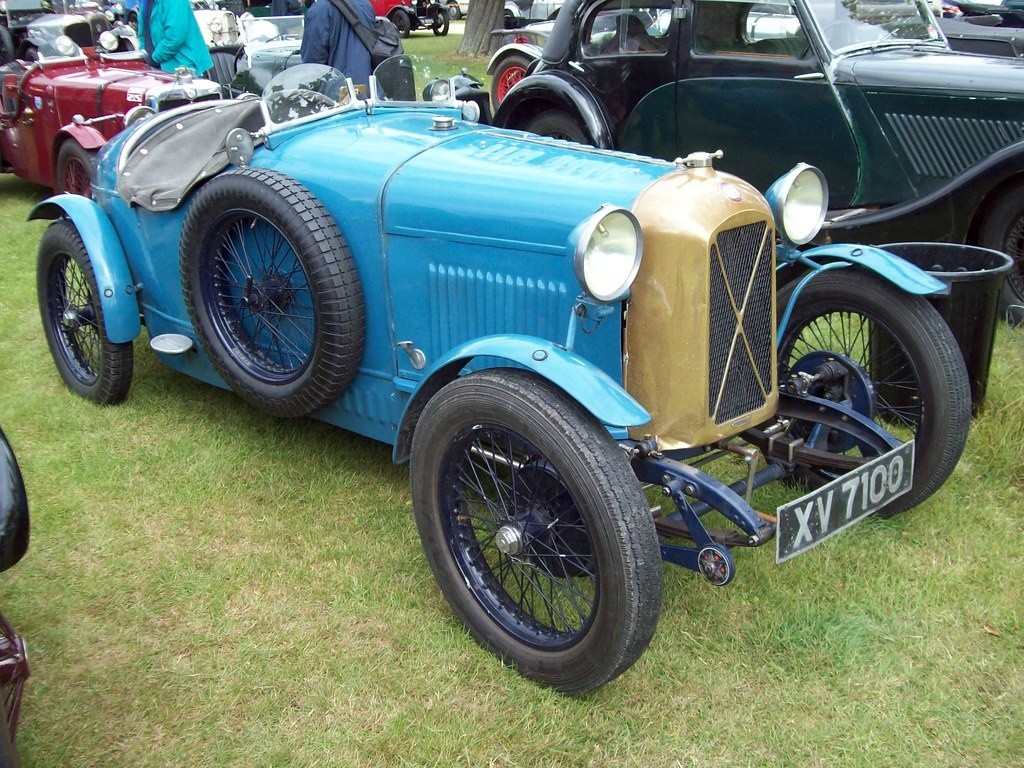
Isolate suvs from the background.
[369,0,451,38]
[486,0,1024,327]
[27,53,971,691]
[0,0,305,200]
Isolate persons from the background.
[298,0,388,103]
[271,0,303,35]
[137,0,214,79]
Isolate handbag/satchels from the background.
[370,15,404,73]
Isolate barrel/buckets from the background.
[867,243,1013,425]
